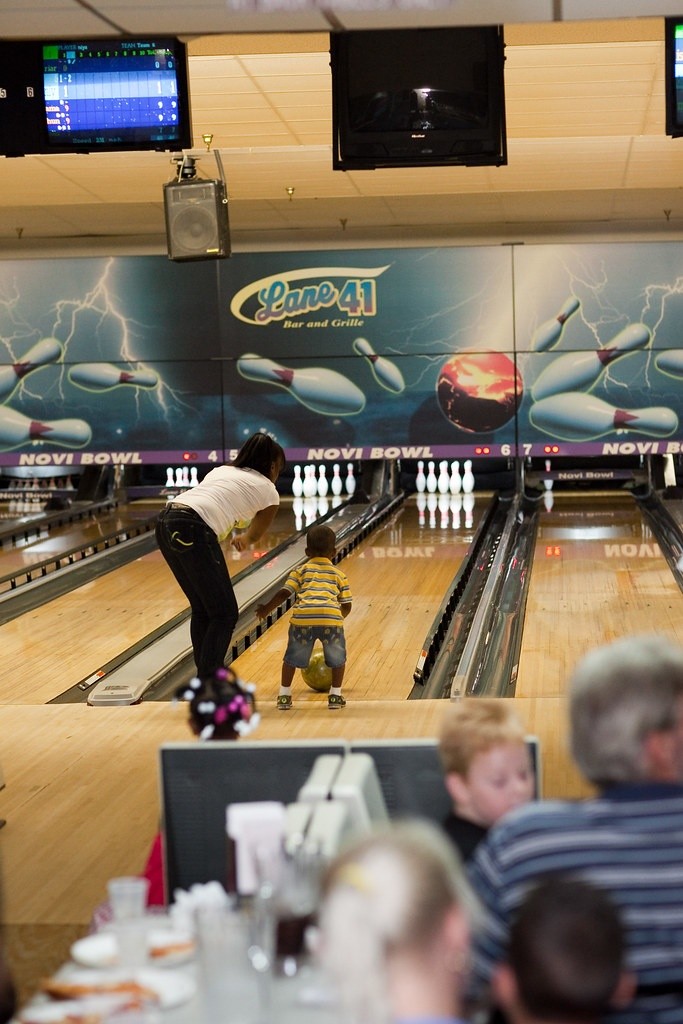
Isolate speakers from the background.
[164,179,232,263]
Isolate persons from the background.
[155,433,286,676]
[255,528,353,710]
[304,635,682,1024]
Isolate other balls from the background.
[300,645,333,692]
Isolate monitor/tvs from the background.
[331,24,508,170]
[1,33,192,158]
[663,16,683,140]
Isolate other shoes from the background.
[276,695,293,710]
[327,694,346,709]
